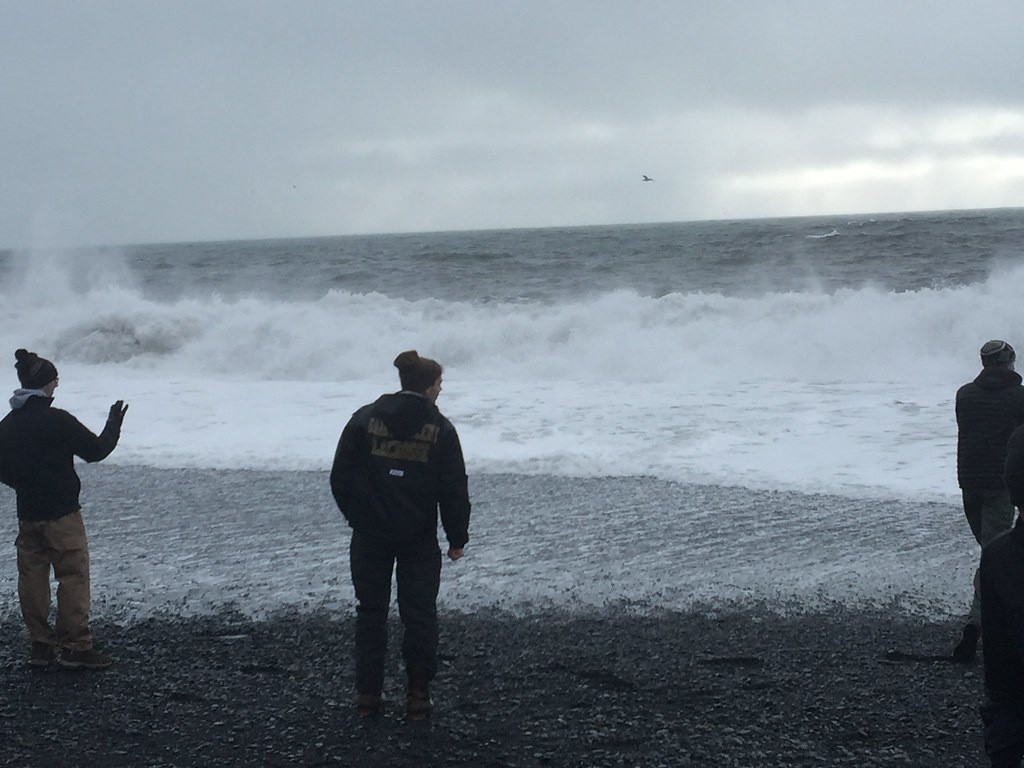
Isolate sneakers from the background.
[358,689,384,710]
[56,646,113,669]
[953,614,979,665]
[29,642,53,668]
[408,694,434,719]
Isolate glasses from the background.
[48,377,59,383]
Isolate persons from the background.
[0,349,127,668]
[979,425,1024,768]
[329,350,471,718]
[952,340,1024,660]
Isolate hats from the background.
[1006,424,1024,504]
[14,349,59,389]
[981,342,1016,367]
[395,350,443,389]
